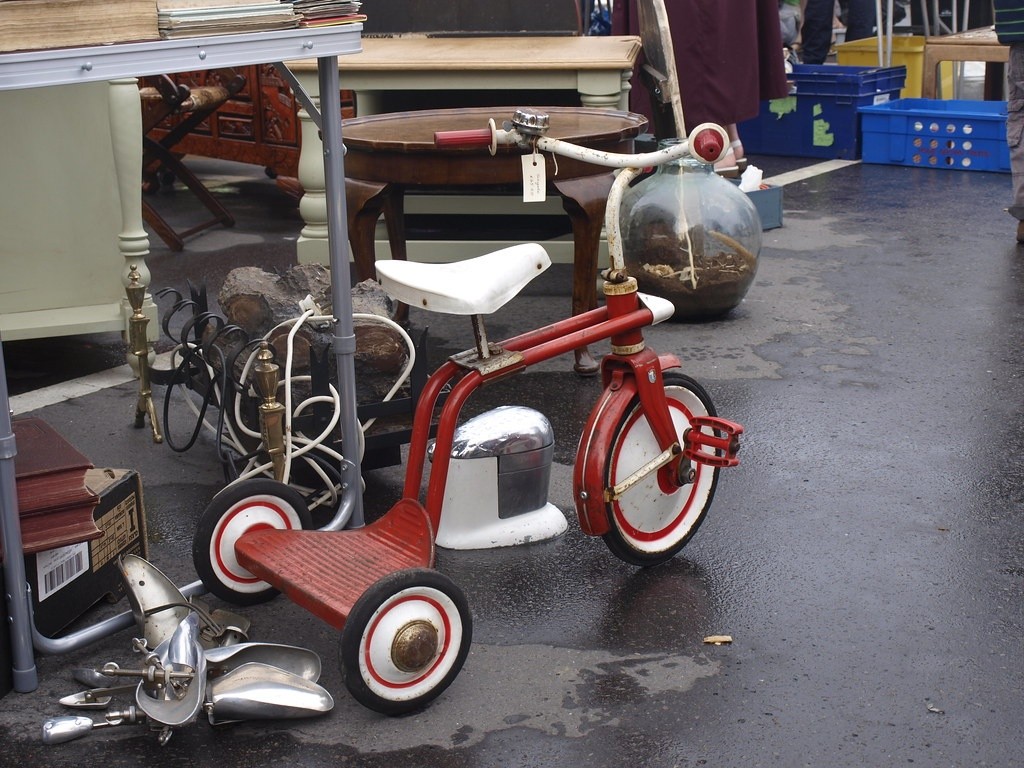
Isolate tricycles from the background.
[190,106,745,717]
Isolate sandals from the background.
[713,147,740,178]
[730,141,749,172]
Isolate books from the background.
[11,416,105,555]
[299,14,367,28]
[0,0,304,51]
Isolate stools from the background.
[921,24,1011,101]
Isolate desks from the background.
[319,37,651,374]
[0,23,365,692]
[321,106,649,371]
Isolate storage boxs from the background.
[0,468,148,664]
[725,179,783,229]
[736,65,908,160]
[831,36,953,99]
[857,98,1012,172]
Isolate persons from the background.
[994,0,1024,241]
[801,0,876,65]
[609,0,789,179]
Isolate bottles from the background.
[621,138,762,318]
